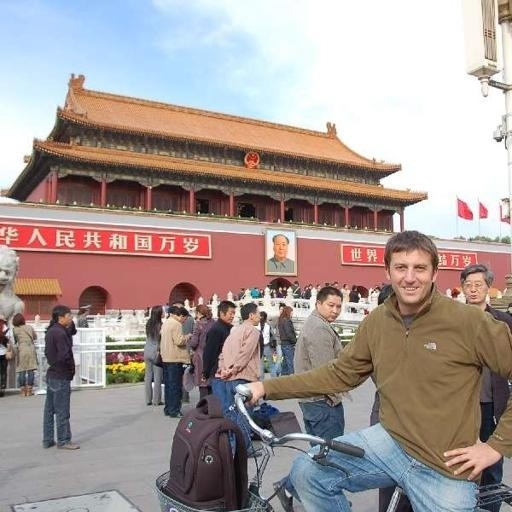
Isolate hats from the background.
[79,305,91,311]
[168,306,178,313]
[0,315,7,322]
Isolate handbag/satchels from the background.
[269,325,277,348]
[6,343,14,360]
[153,334,162,368]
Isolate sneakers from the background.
[57,442,80,450]
[43,441,56,448]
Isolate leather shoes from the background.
[175,412,184,418]
[273,481,294,512]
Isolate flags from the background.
[456,195,473,220]
[500,202,510,224]
[478,197,488,218]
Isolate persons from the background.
[279,302,285,315]
[267,234,294,273]
[218,303,262,457]
[325,283,330,287]
[0,314,14,397]
[77,304,91,328]
[178,307,194,403]
[44,318,77,347]
[277,287,283,298]
[187,304,216,400]
[166,306,178,320]
[340,284,350,302]
[278,306,297,375]
[505,303,512,315]
[316,284,321,291]
[42,305,80,449]
[283,288,287,297]
[144,305,165,406]
[304,284,312,299]
[370,285,409,512]
[292,281,301,308]
[13,313,37,395]
[256,311,278,381]
[273,286,352,512]
[160,307,193,417]
[349,285,360,313]
[202,300,237,440]
[244,231,511,512]
[460,264,512,512]
[330,281,339,288]
[302,286,308,299]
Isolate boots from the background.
[19,387,26,396]
[26,386,34,396]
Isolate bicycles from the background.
[154,384,512,511]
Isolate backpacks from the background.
[165,395,248,510]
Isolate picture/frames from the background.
[265,228,298,277]
